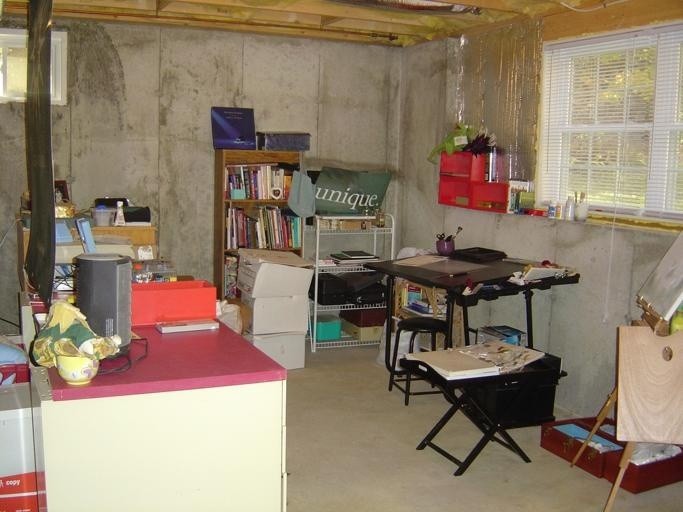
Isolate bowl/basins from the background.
[53,355,101,386]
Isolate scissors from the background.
[436,233,445,241]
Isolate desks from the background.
[362,256,585,359]
[396,352,556,476]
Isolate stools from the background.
[390,316,445,398]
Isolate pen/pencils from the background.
[449,272,470,278]
[445,226,463,242]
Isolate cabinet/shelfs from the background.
[13,210,158,293]
[17,305,291,510]
[214,149,304,337]
[304,214,394,354]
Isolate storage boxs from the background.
[239,332,303,372]
[237,293,310,335]
[309,314,341,342]
[438,149,487,179]
[469,179,527,215]
[601,438,681,494]
[230,249,312,299]
[342,319,384,342]
[537,411,628,477]
[436,173,472,210]
[124,277,220,332]
[339,308,388,328]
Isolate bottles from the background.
[548,196,575,221]
[501,144,527,180]
[669,307,683,336]
[114,201,125,226]
[362,207,386,229]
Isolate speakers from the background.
[75,253,132,349]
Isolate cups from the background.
[436,240,455,254]
[574,203,589,222]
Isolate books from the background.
[223,162,302,298]
[154,318,220,334]
[307,251,382,271]
[436,371,502,381]
[514,190,536,216]
[479,324,525,341]
[505,177,530,215]
[397,282,447,321]
[53,263,78,292]
[124,220,151,227]
[447,340,544,373]
[400,347,498,376]
